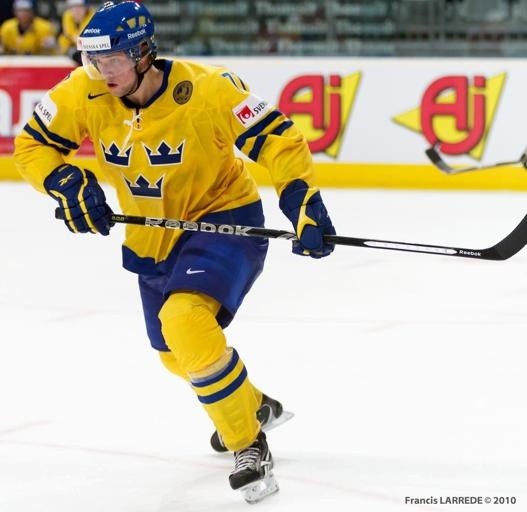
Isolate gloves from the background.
[279,180,336,259]
[44,164,116,236]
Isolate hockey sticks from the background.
[424,145,522,174]
[54,205,526,260]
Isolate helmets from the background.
[76,1,157,82]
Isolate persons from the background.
[10,0,335,489]
[0,0,57,55]
[57,0,98,65]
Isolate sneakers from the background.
[211,394,283,453]
[230,431,274,490]
